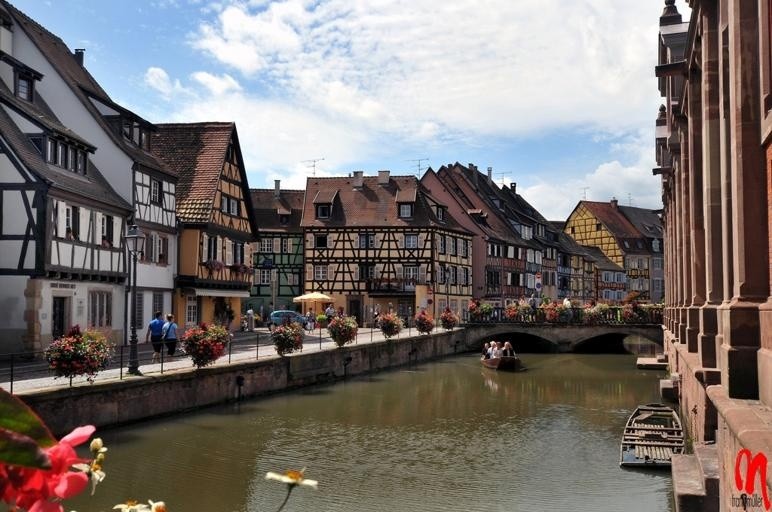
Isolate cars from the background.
[266,311,307,331]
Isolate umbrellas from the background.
[292,292,336,320]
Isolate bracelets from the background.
[177,340,180,342]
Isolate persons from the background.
[481,340,515,360]
[305,307,317,335]
[519,294,526,306]
[145,310,165,364]
[325,303,336,324]
[563,293,572,308]
[528,293,537,309]
[162,313,183,362]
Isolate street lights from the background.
[122,223,147,375]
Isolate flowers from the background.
[376,311,407,338]
[45,324,120,385]
[271,321,307,356]
[414,310,435,334]
[327,314,359,346]
[180,321,235,369]
[464,294,666,326]
[439,305,458,329]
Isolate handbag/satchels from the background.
[161,332,170,342]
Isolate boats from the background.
[618,401,686,469]
[479,355,523,372]
